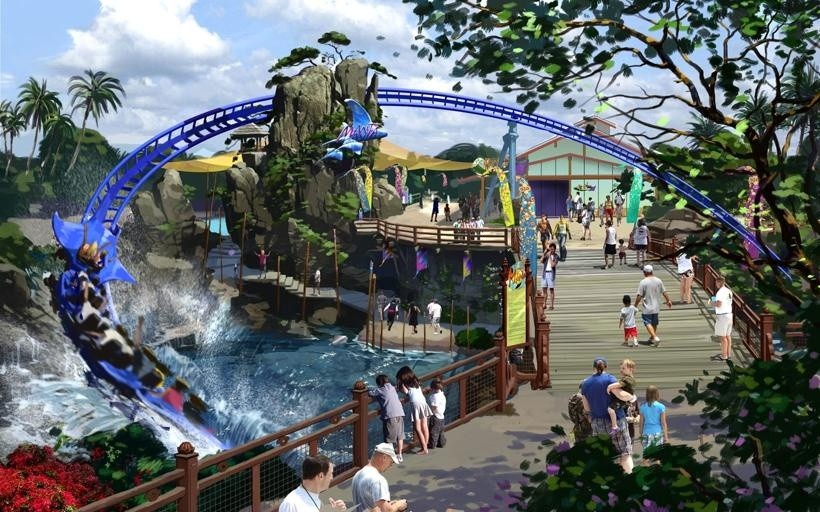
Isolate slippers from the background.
[543,305,554,310]
[714,354,728,360]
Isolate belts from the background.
[546,271,552,273]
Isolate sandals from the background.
[608,427,620,437]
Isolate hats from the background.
[644,264,653,273]
[592,356,608,368]
[374,442,400,464]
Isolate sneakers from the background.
[633,340,640,348]
[416,449,428,456]
[648,336,661,347]
[398,453,404,462]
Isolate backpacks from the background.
[568,401,581,423]
